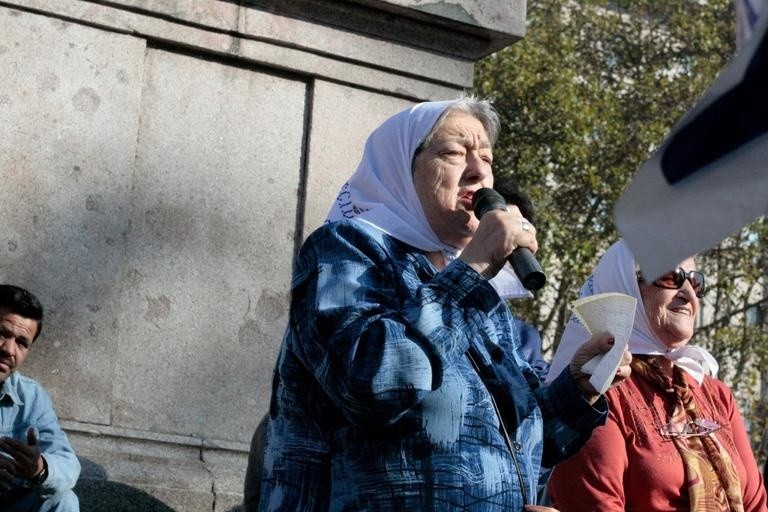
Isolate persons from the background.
[243,93,635,511]
[0,283,82,511]
[543,237,768,512]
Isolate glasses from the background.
[657,415,725,441]
[637,266,707,297]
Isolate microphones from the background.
[471,185,546,291]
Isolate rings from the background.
[521,222,530,232]
